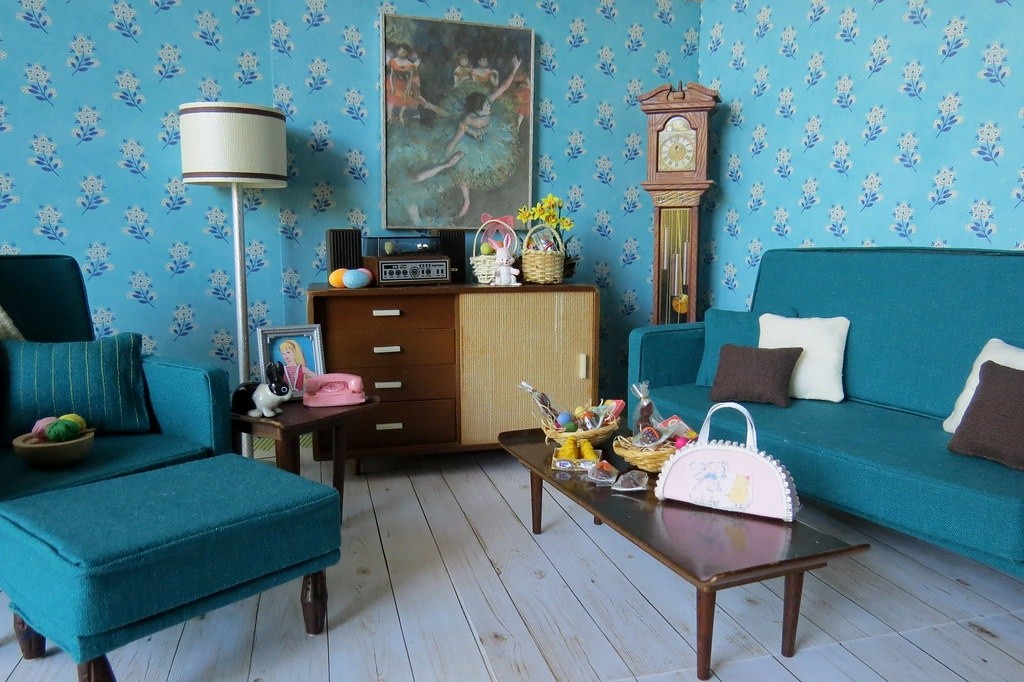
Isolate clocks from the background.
[638,80,722,326]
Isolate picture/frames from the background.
[256,323,327,401]
[379,12,535,231]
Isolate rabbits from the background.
[488,233,523,286]
[231,360,294,416]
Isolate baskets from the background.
[522,224,565,285]
[613,436,699,472]
[542,418,621,447]
[471,220,518,285]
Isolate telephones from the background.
[303,373,365,407]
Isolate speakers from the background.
[432,229,465,286]
[326,229,361,284]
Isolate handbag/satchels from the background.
[655,402,800,521]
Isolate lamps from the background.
[179,101,288,457]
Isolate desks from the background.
[230,396,381,525]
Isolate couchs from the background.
[628,246,1024,584]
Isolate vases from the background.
[514,256,577,279]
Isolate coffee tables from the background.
[498,428,871,679]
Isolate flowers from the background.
[517,193,579,259]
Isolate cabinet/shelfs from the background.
[306,293,600,476]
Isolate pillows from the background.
[708,343,803,409]
[695,308,798,386]
[942,337,1024,433]
[946,360,1024,471]
[0,332,158,441]
[758,313,851,404]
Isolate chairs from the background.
[0,254,231,507]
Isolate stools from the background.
[0,451,342,682]
